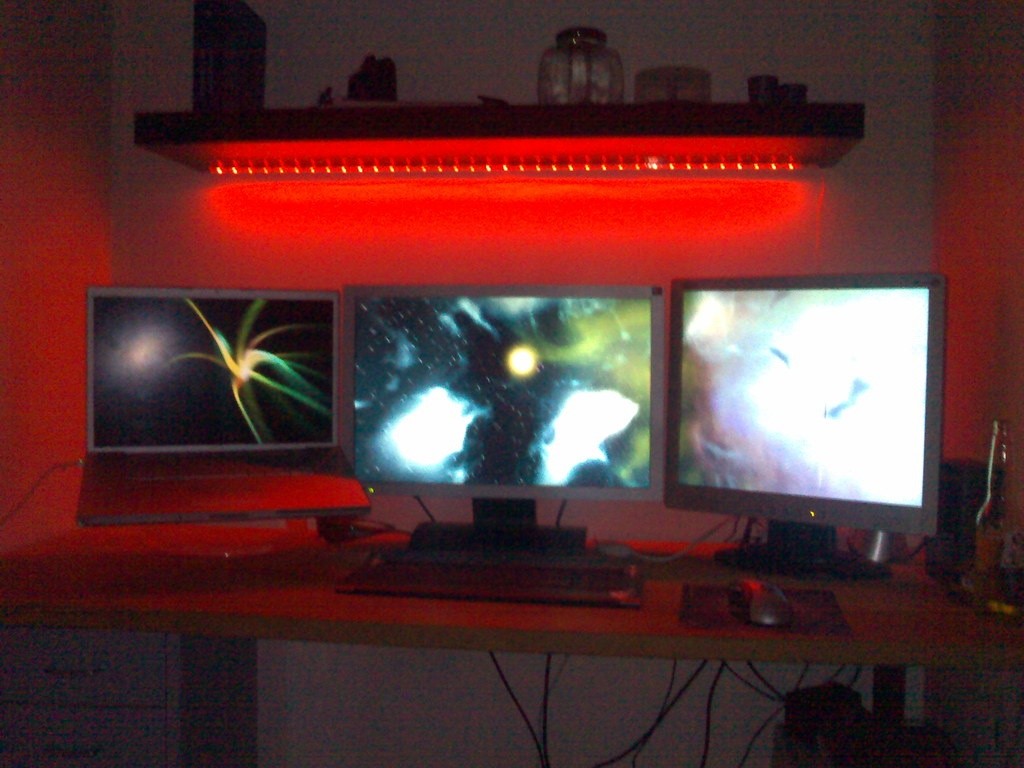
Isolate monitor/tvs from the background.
[342,286,669,557]
[667,273,944,580]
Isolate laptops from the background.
[76,284,370,528]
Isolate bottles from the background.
[977,416,1024,613]
[538,24,623,107]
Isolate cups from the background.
[776,82,808,106]
[926,460,1002,575]
[747,75,779,106]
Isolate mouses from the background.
[732,578,793,628]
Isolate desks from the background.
[0,538,1024,768]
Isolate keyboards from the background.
[339,558,642,607]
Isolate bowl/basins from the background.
[632,64,714,99]
